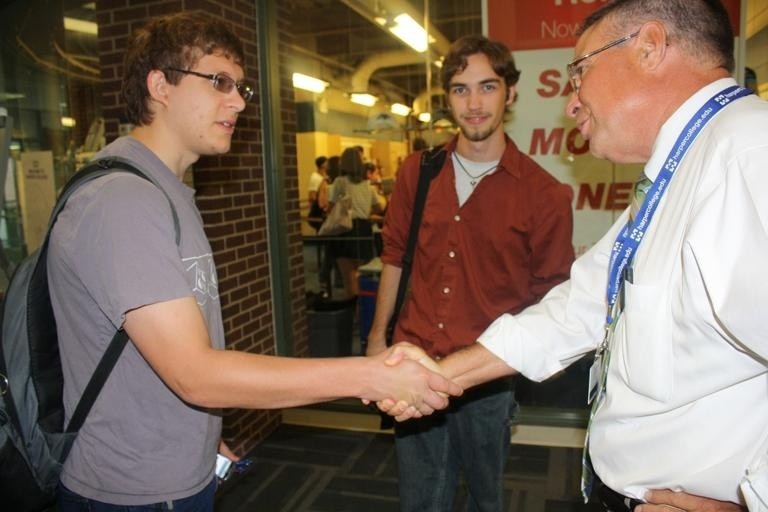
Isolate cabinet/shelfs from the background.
[356,256,385,356]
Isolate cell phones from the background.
[214,453,237,482]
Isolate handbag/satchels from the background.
[318,193,353,235]
[308,201,327,231]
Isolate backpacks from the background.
[2,156,180,498]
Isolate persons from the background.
[308,135,429,299]
[362,1,768,510]
[44,11,468,512]
[364,34,572,511]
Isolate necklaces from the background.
[453,150,497,186]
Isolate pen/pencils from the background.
[625,268,633,284]
[235,459,254,468]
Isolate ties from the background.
[581,179,650,502]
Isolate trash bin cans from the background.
[305,300,355,357]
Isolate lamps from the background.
[292,70,432,124]
[389,12,437,54]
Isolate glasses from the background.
[163,65,253,101]
[566,32,638,94]
